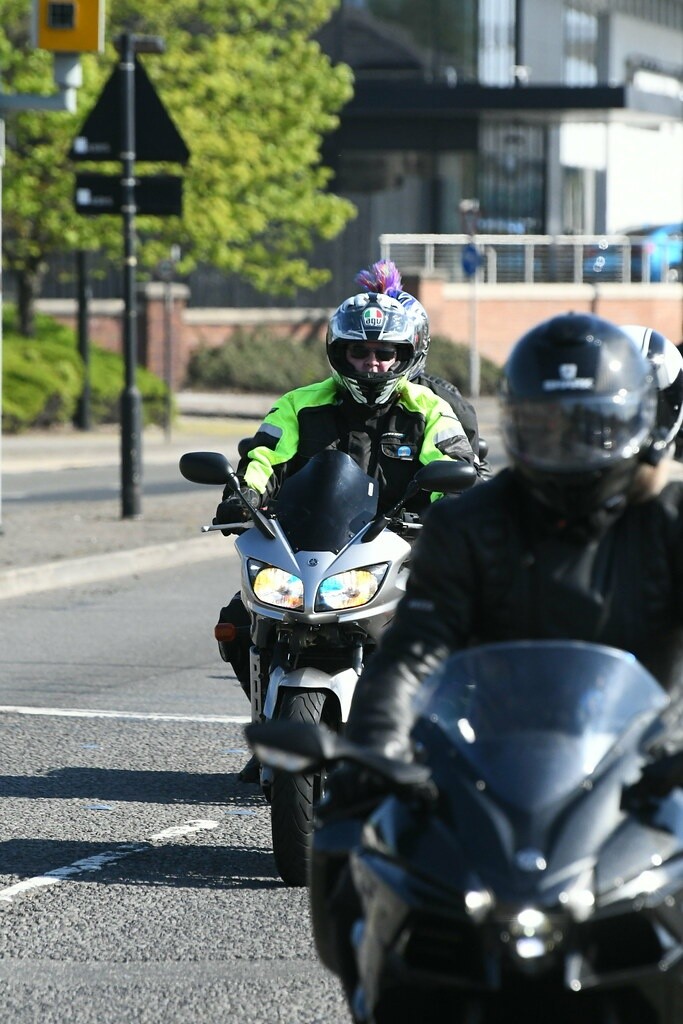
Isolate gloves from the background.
[311,768,393,830]
[216,490,262,536]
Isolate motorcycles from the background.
[177,451,483,886]
[248,634,683,1024]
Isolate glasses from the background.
[347,343,403,363]
[505,417,621,458]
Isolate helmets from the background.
[499,311,656,507]
[352,260,430,381]
[618,324,683,452]
[324,293,419,409]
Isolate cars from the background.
[566,221,683,285]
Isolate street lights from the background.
[111,33,163,518]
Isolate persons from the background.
[322,312,683,1024]
[215,292,476,784]
[356,259,480,470]
[620,326,683,462]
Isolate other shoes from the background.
[232,748,262,783]
